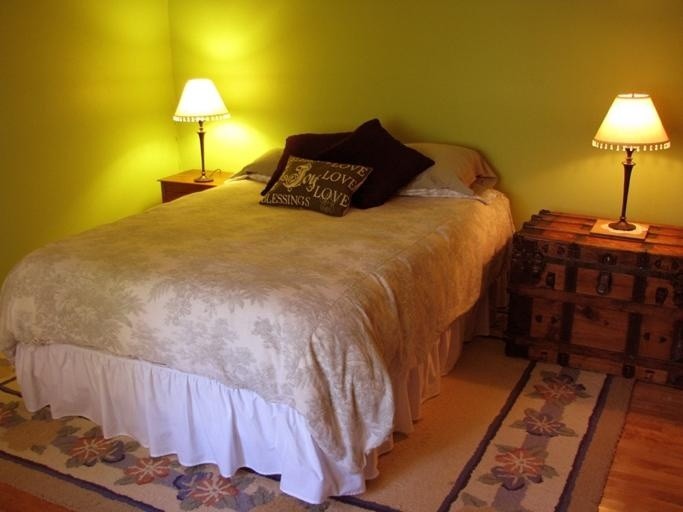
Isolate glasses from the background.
[171,78,228,183]
[592,93,671,232]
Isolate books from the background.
[503,209,683,393]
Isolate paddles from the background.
[0,337,634,512]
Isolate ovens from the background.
[0,181,517,506]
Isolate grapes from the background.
[156,167,232,204]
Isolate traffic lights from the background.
[223,116,498,217]
